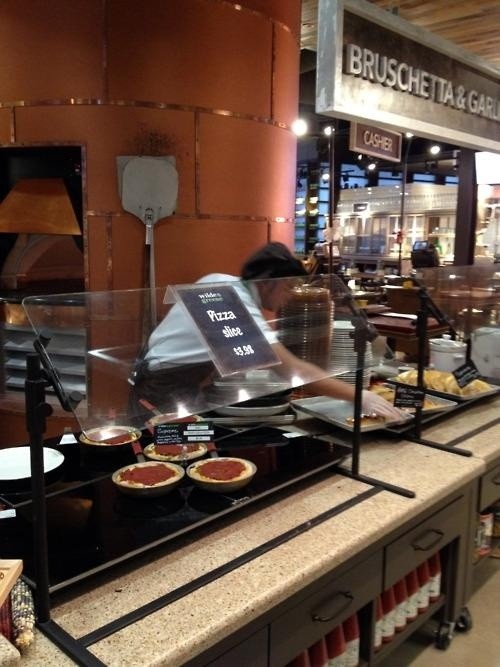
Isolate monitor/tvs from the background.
[412,240,429,253]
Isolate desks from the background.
[339,254,411,276]
[333,309,456,364]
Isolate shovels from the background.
[116,156,178,352]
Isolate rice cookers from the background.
[425,333,468,370]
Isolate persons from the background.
[127,242,404,438]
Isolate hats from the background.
[240,241,310,280]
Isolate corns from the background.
[0,578,37,649]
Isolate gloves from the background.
[361,389,405,424]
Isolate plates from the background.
[1,411,260,494]
[276,284,335,398]
[330,320,374,391]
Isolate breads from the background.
[347,369,492,427]
